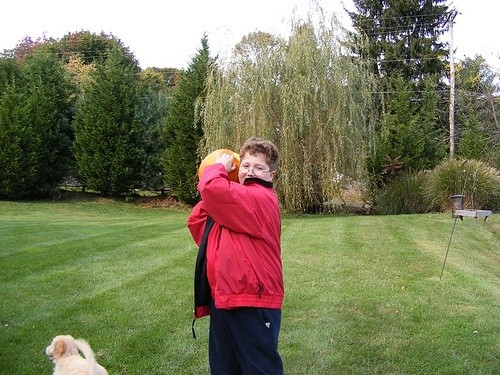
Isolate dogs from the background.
[45,334,109,375]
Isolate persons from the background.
[185,136,287,375]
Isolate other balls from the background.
[199,149,240,183]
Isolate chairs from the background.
[451,195,492,221]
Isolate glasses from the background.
[238,166,274,175]
